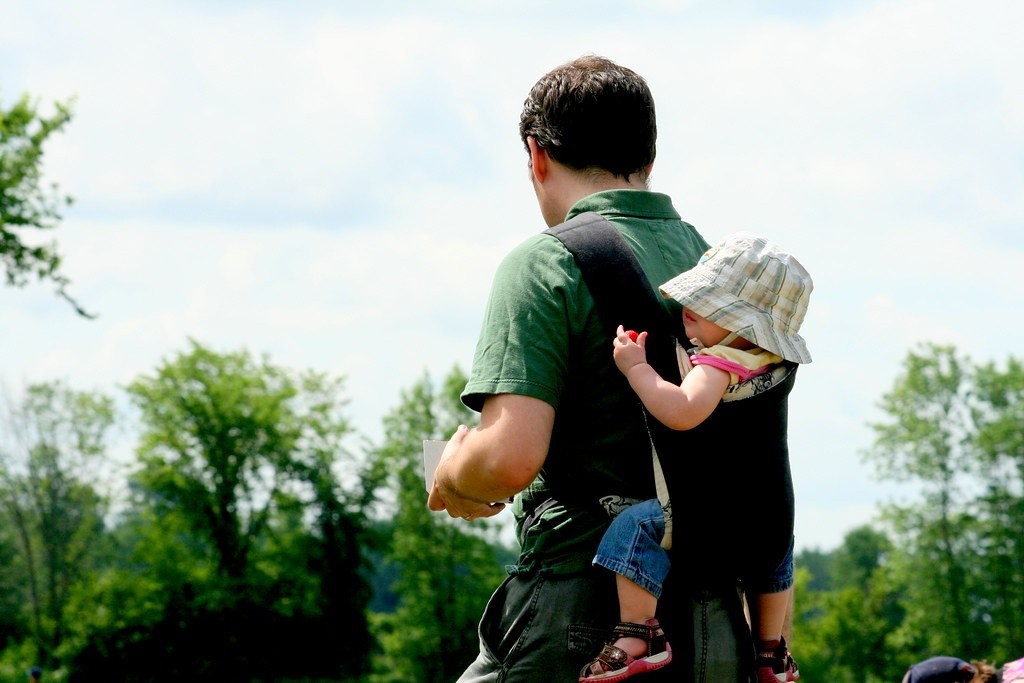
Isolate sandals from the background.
[578,618,673,683]
[755,635,800,683]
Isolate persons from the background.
[901,657,1024,683]
[424,55,767,683]
[579,234,814,683]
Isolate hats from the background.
[901,657,974,683]
[659,234,813,364]
